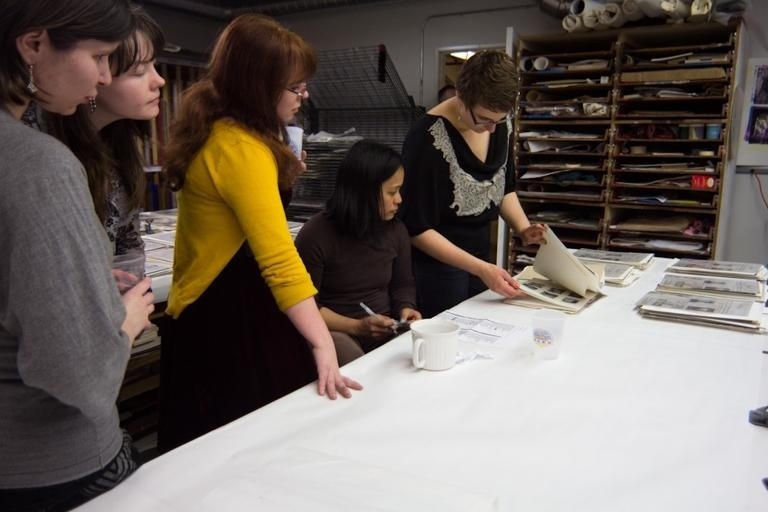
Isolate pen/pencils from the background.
[360,302,399,334]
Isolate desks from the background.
[76,251,768,511]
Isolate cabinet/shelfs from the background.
[507,15,750,278]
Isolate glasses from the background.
[285,85,308,96]
[470,109,510,126]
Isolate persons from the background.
[294,139,422,367]
[1,2,165,512]
[158,12,364,454]
[438,84,457,104]
[398,48,548,322]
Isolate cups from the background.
[410,318,462,371]
[688,123,722,141]
[285,126,304,161]
[531,309,565,361]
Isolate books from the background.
[504,225,768,333]
